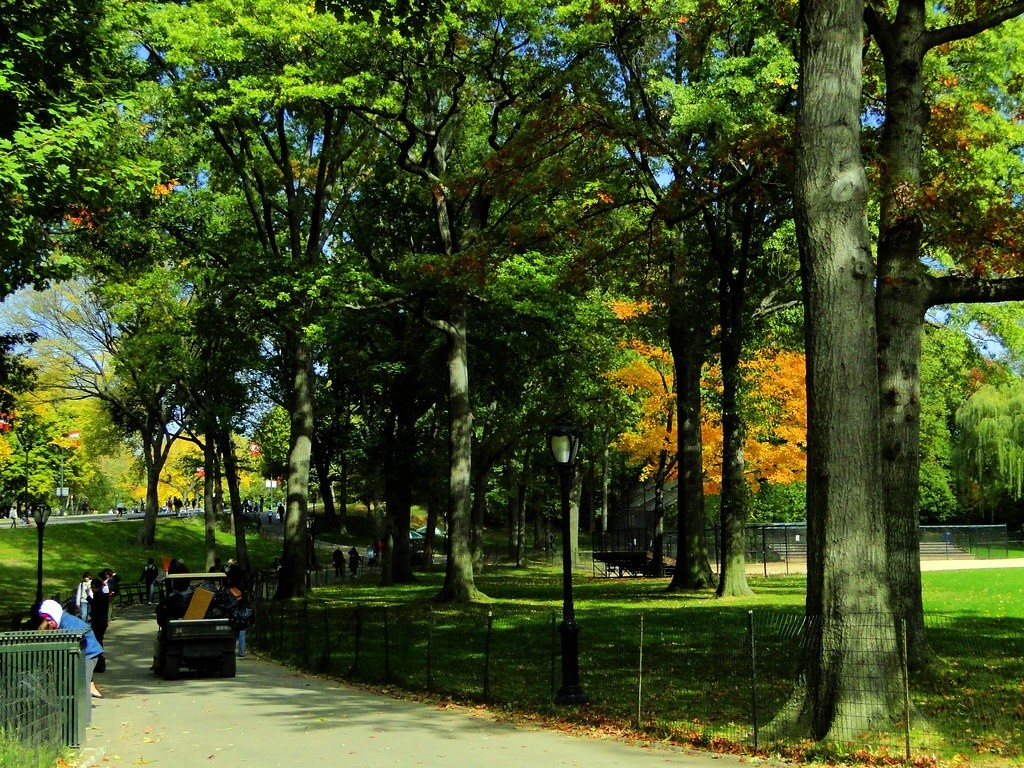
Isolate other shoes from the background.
[89,681,102,697]
[148,601,152,605]
[93,658,106,673]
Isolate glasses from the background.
[90,586,97,589]
[104,574,108,575]
[45,618,52,622]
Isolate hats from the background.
[228,558,234,564]
[177,559,185,563]
[230,588,242,597]
[148,558,155,564]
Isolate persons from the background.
[0,493,385,709]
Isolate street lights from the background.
[31,501,52,607]
[545,416,590,707]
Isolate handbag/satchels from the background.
[368,549,375,559]
[73,582,83,608]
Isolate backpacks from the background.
[146,566,155,582]
[214,565,223,573]
[111,583,119,596]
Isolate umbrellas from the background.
[409,530,424,540]
[417,524,445,538]
